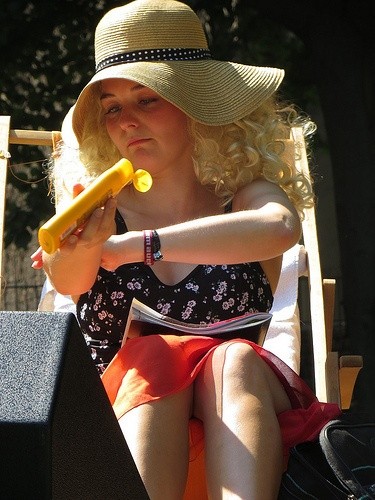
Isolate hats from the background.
[71,0,286,151]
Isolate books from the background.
[120,297,273,350]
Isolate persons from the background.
[29,39,316,496]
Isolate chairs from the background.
[1,115,335,402]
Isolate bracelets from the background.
[141,229,155,266]
[151,230,163,262]
